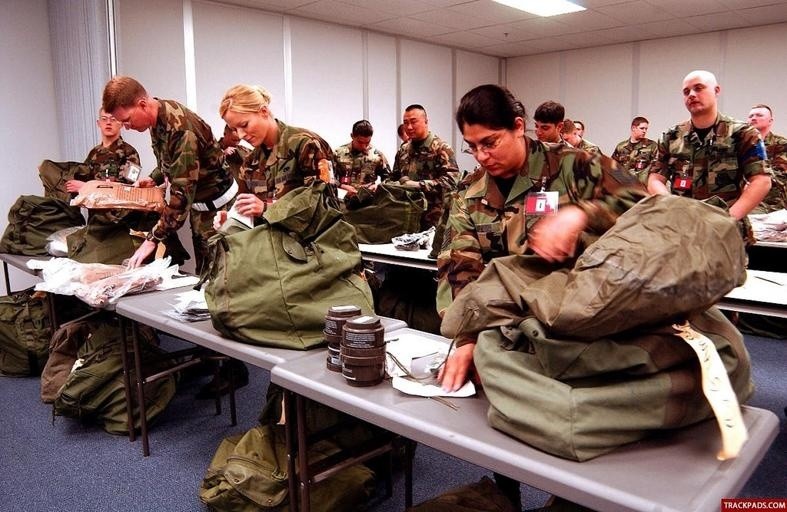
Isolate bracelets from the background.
[146,232,161,244]
[259,198,268,220]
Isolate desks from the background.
[0,210,787,511]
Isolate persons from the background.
[212,83,344,243]
[102,77,250,400]
[434,83,654,511]
[648,69,773,326]
[60,106,142,224]
[335,103,786,233]
[216,124,255,177]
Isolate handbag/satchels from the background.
[0,284,91,381]
[197,421,382,512]
[0,158,136,267]
[436,192,758,470]
[343,180,429,246]
[193,196,381,352]
[38,310,179,436]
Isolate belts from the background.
[191,176,240,212]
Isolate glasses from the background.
[460,126,511,154]
[117,105,139,126]
